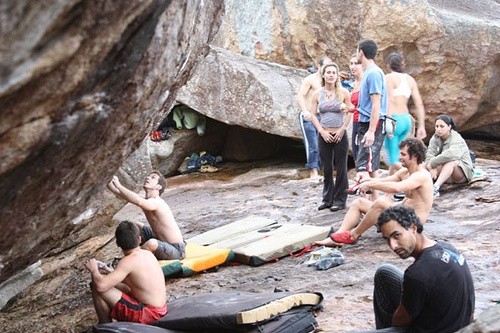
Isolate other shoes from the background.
[318,203,331,210]
[330,205,344,211]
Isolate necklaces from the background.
[323,90,338,99]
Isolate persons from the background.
[310,64,352,211]
[374,205,475,333]
[342,52,364,164]
[314,138,433,245]
[386,52,426,176]
[356,39,387,202]
[107,170,186,260]
[298,58,333,182]
[427,116,473,197]
[84,220,167,324]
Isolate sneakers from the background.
[434,188,440,197]
[345,180,372,194]
[330,231,359,244]
[177,150,222,173]
[394,191,406,199]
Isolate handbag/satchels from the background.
[382,115,397,137]
